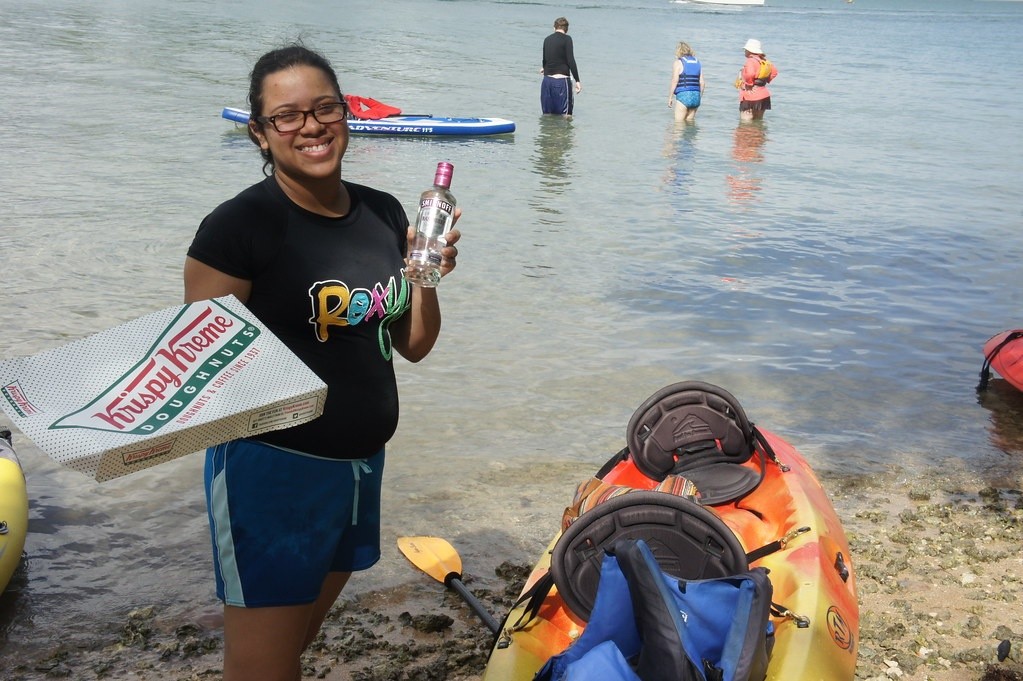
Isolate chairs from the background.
[551,499,772,680]
[625,375,778,508]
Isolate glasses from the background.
[255,101,348,135]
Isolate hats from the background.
[742,39,764,54]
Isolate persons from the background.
[739,39,777,121]
[540,17,581,114]
[668,42,704,122]
[184,46,461,681]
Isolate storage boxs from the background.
[0,293,329,483]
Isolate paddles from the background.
[395,536,501,634]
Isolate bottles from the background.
[403,162,457,288]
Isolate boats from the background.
[479,379,860,681]
[982,329,1023,394]
[221,107,514,137]
[0,434,29,604]
